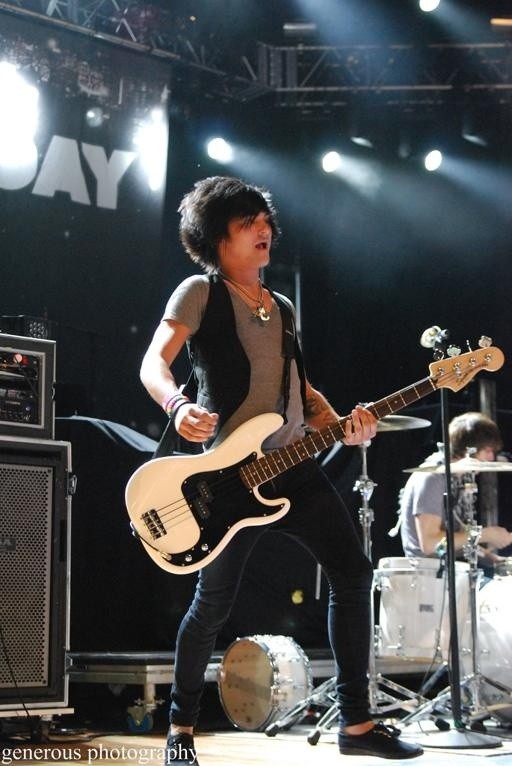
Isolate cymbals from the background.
[401,457,512,475]
[335,412,432,434]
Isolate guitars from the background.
[123,332,506,577]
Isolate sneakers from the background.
[338,724,423,759]
[165,729,200,766]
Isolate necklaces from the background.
[217,267,273,323]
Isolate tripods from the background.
[308,433,419,747]
[266,440,432,738]
[383,466,512,730]
[437,476,510,715]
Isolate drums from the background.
[214,632,316,731]
[373,554,472,669]
[450,572,511,730]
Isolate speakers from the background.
[0,437,72,707]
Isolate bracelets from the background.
[158,392,191,415]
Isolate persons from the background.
[139,173,426,763]
[394,408,512,573]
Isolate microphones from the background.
[496,451,511,461]
[419,326,449,348]
